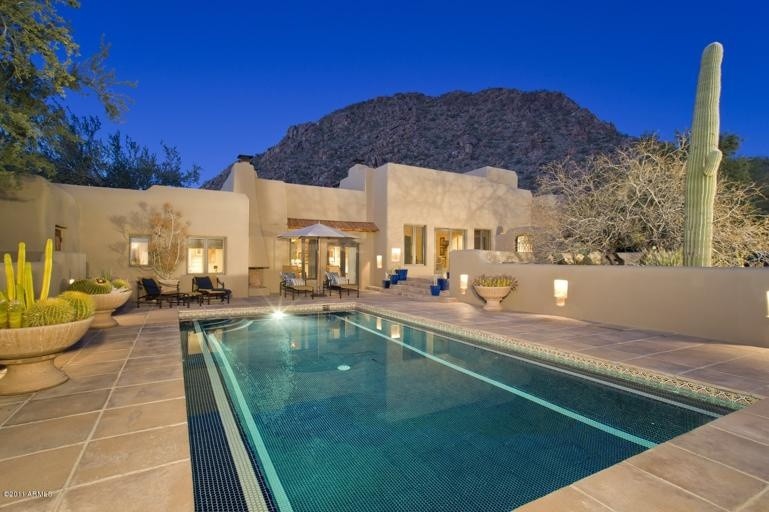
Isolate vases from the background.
[474,285,511,311]
[430,279,448,296]
[383,269,407,288]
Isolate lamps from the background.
[554,279,567,306]
[459,275,469,295]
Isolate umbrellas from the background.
[276,220,361,293]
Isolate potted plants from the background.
[0,239,133,396]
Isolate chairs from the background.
[136,275,232,308]
[280,264,359,300]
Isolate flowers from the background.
[470,274,517,288]
